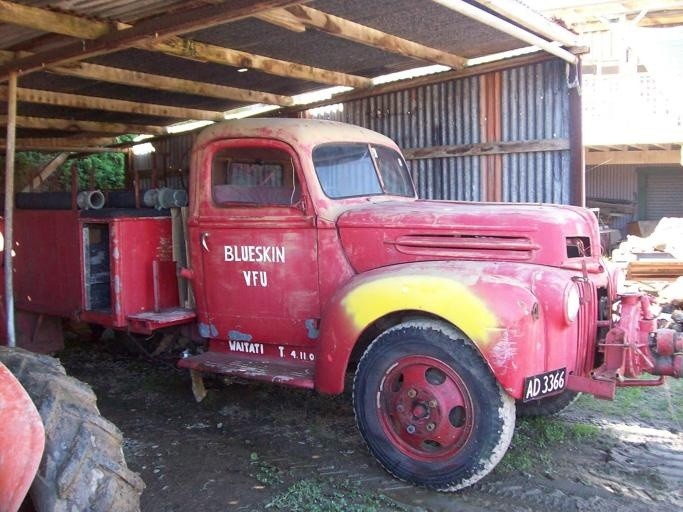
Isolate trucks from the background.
[1,116,682,492]
